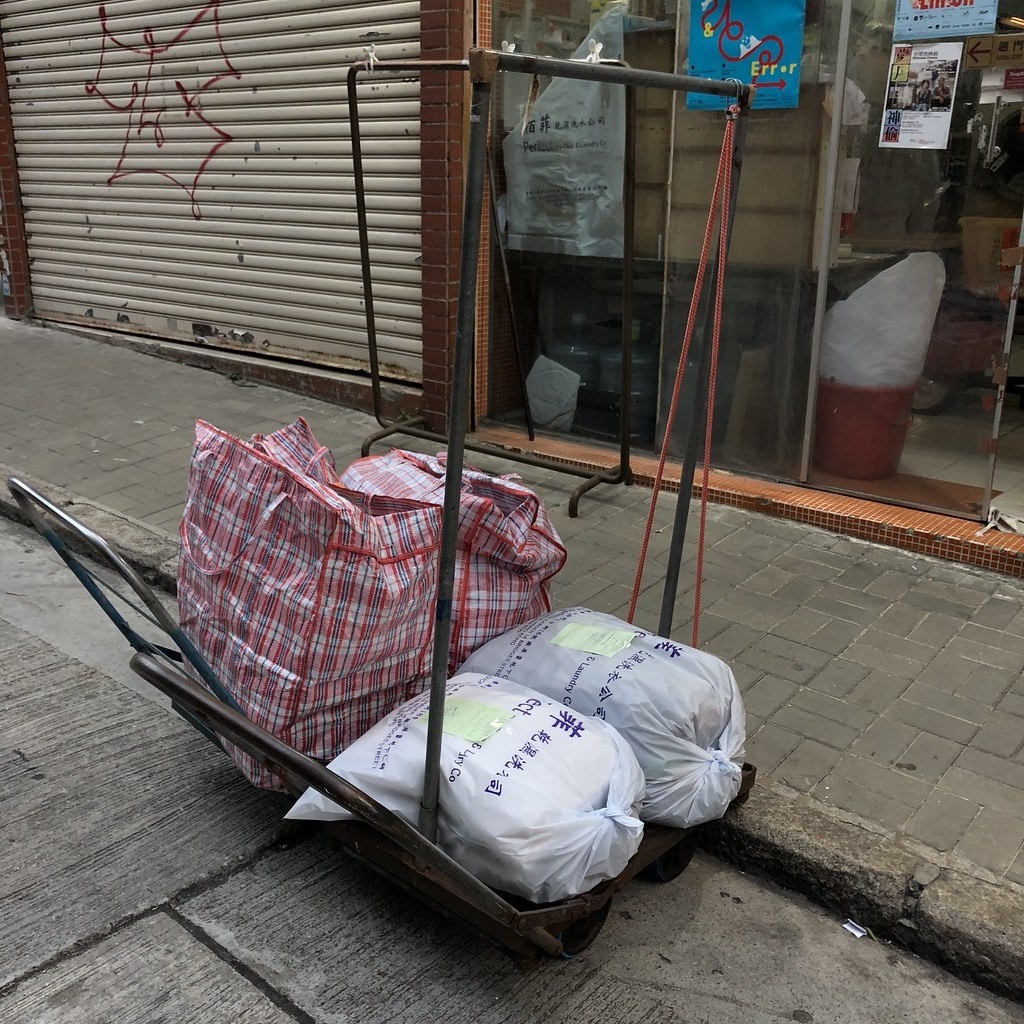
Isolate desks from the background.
[519,254,897,459]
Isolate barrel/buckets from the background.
[958,216,1022,297]
[813,377,920,482]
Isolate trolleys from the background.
[2,45,761,966]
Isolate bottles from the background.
[600,320,658,433]
[549,313,599,416]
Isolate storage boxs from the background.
[958,216,1022,281]
